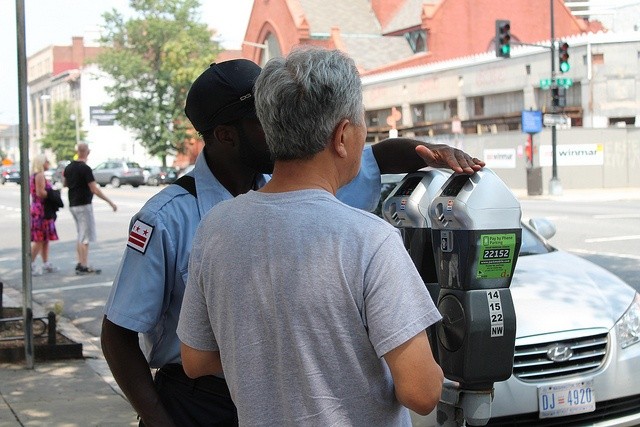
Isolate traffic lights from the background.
[559,41,570,71]
[495,19,510,57]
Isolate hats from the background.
[184,57,262,134]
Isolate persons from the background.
[30,153,63,274]
[99,58,485,427]
[63,143,118,274]
[177,42,446,427]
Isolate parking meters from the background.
[381,166,522,426]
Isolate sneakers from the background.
[31,265,43,277]
[79,267,102,276]
[42,263,60,273]
[74,263,82,274]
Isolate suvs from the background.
[92,161,143,187]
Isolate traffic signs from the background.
[540,78,572,89]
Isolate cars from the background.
[147,168,177,186]
[377,173,640,426]
[0,166,21,183]
